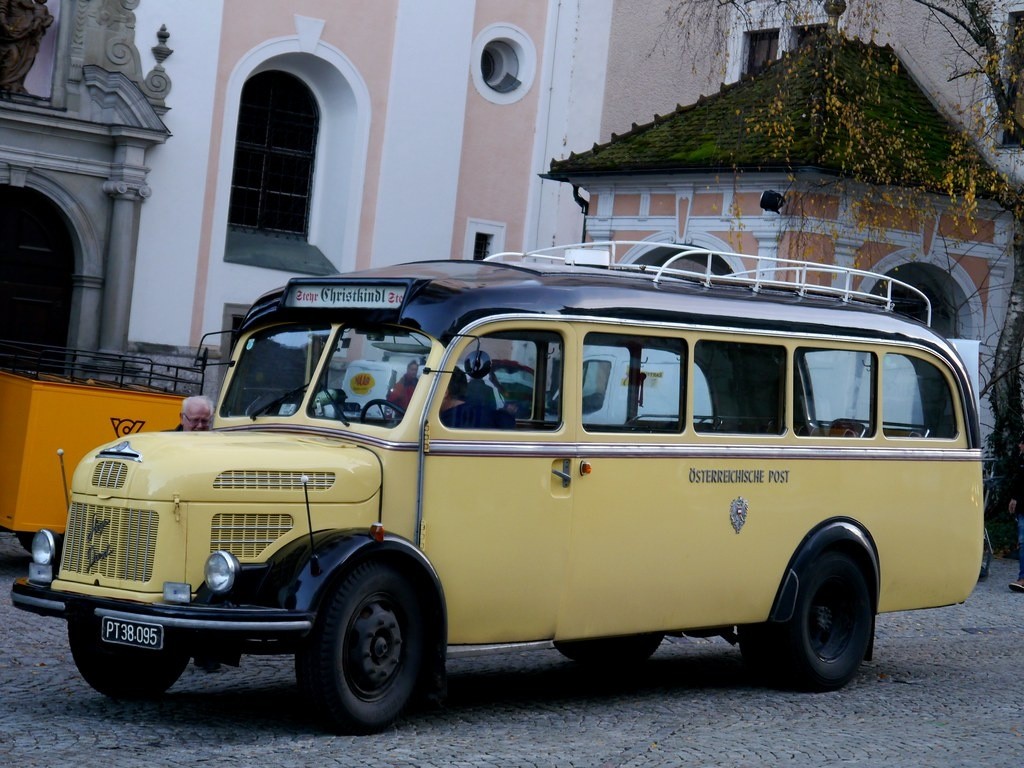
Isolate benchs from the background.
[623,413,783,435]
[793,418,866,438]
[833,419,932,438]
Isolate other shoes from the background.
[1008,582,1024,592]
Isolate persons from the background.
[436,363,494,429]
[161,395,216,431]
[0,0,54,93]
[387,361,418,424]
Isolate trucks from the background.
[339,329,983,437]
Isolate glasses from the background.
[182,413,210,426]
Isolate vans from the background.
[10,241,984,737]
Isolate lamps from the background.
[759,189,785,215]
